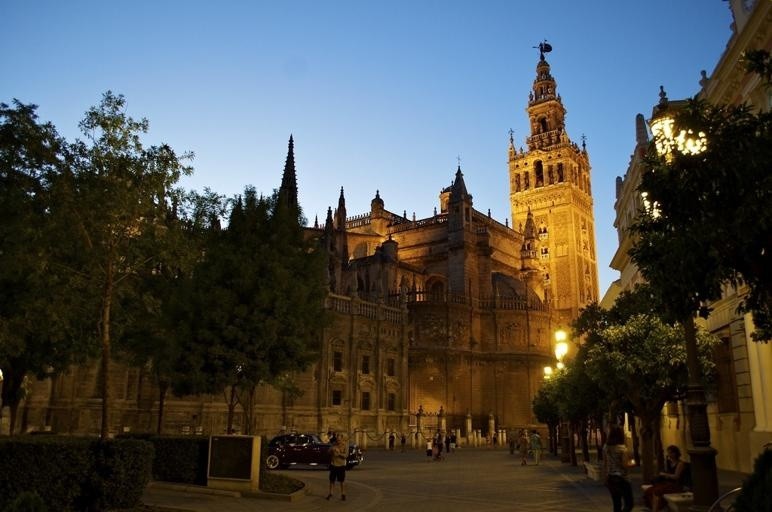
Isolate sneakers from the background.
[340,494,347,500]
[326,494,334,500]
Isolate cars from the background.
[265,433,364,472]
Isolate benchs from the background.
[640,484,694,512]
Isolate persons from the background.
[230,428,236,435]
[326,432,349,501]
[322,425,545,467]
[639,444,687,512]
[599,426,638,511]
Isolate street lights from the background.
[555,328,575,462]
[634,83,725,511]
[542,365,560,456]
